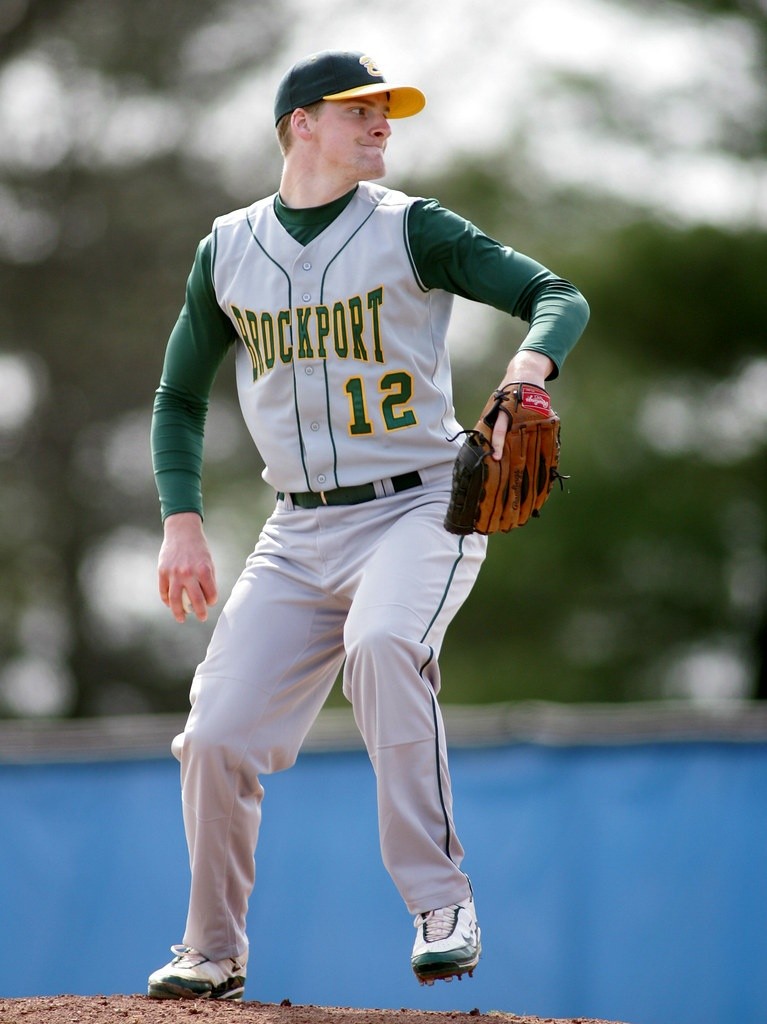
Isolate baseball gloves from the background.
[442,380,574,536]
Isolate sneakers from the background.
[410,896,482,986]
[148,943,248,1003]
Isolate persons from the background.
[149,46,590,1002]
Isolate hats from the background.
[273,48,427,128]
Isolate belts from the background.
[277,471,423,509]
